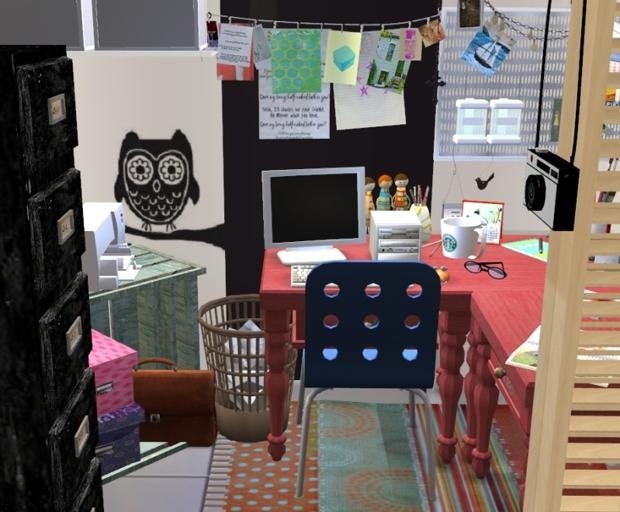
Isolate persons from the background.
[365,172,412,235]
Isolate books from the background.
[501,238,549,263]
[505,325,620,388]
